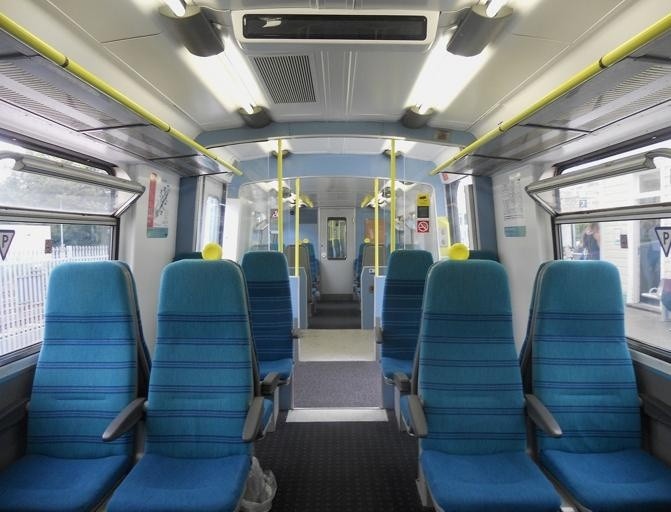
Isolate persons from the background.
[581,219,600,260]
[642,221,661,293]
